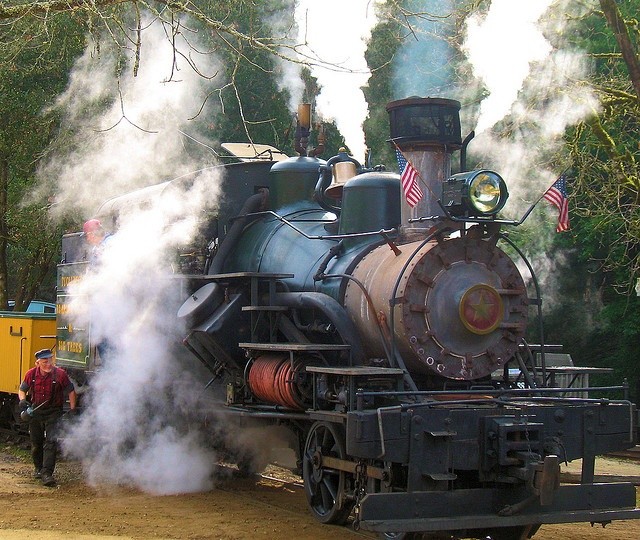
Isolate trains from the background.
[0,94,640,539]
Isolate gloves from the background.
[18,399,31,410]
[67,409,78,421]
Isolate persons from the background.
[80,220,118,269]
[17,346,76,486]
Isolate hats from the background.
[34,349,54,359]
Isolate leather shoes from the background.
[41,473,55,486]
[33,467,41,478]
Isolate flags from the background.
[395,149,424,209]
[544,173,570,233]
